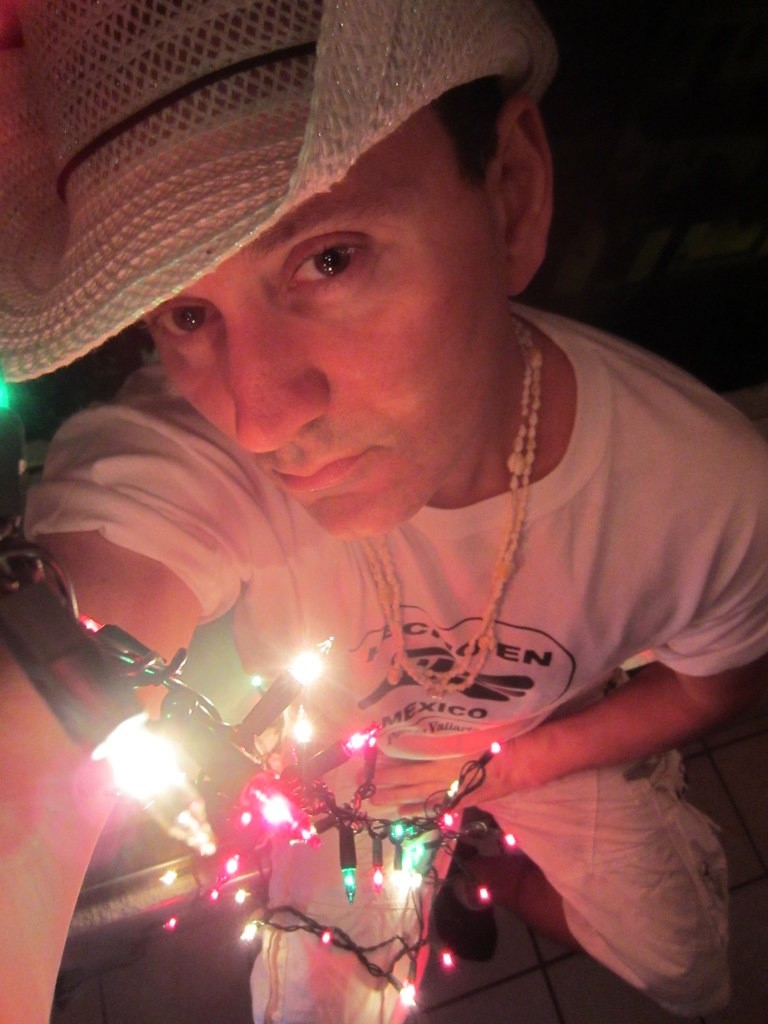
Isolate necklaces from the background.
[357,317,544,698]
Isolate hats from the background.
[0,0,559,385]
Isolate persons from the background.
[0,0,768,1024]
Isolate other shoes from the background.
[432,842,501,964]
